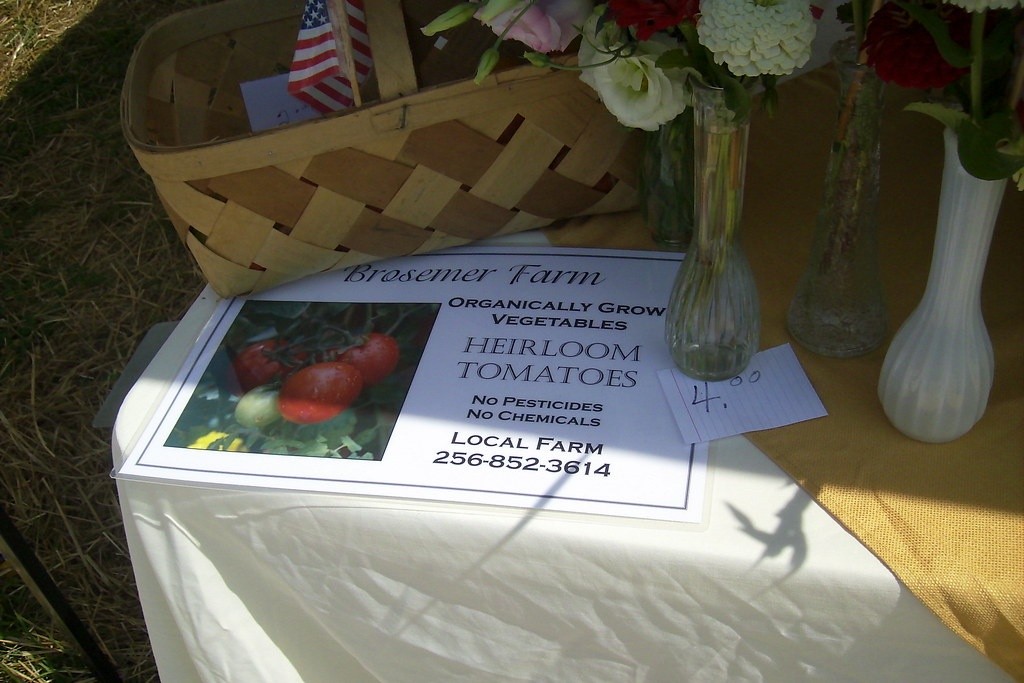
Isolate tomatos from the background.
[232,332,398,428]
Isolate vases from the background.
[786,35,891,359]
[664,72,759,382]
[639,108,700,245]
[877,125,1007,444]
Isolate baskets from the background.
[120,0,644,300]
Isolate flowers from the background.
[417,0,1024,332]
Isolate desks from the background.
[112,0,1024,683]
